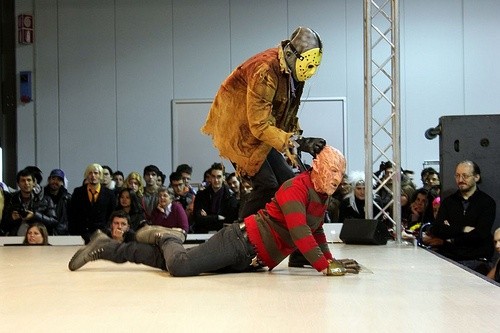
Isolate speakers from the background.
[438,114,500,236]
[339,218,390,245]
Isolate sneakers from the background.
[69,229,113,271]
[136,225,187,244]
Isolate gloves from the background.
[328,257,359,276]
[295,137,326,157]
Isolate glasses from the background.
[454,175,472,179]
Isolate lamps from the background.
[425,122,442,140]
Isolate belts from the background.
[238,221,261,269]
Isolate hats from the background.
[48,169,65,181]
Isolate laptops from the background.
[322,223,343,242]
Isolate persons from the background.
[0,163,253,246]
[325,161,500,283]
[203,27,325,268]
[67,146,359,276]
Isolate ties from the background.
[88,188,97,206]
